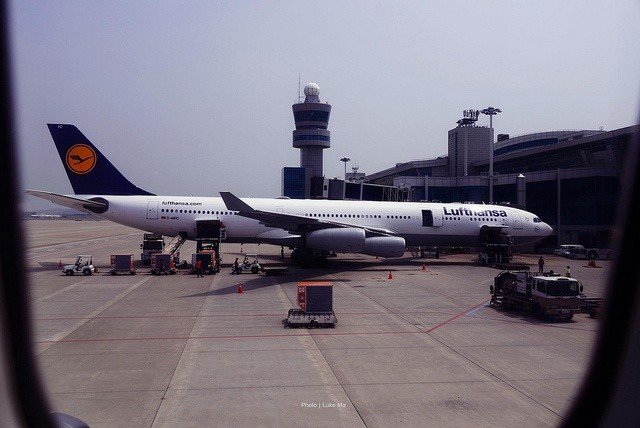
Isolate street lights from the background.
[480,106,503,204]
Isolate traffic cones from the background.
[238,284,244,293]
[388,271,394,280]
[421,263,426,272]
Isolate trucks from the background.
[489,272,584,320]
[141,233,163,264]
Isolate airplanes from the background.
[22,123,553,265]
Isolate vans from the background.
[554,245,584,256]
